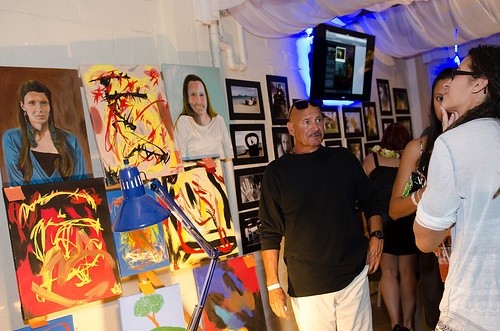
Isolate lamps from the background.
[111,166,220,331]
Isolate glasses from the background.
[288,98,323,120]
[451,66,487,94]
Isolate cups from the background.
[438,247,451,282]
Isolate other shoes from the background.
[392,324,409,331]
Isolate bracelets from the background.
[267,283,282,292]
[411,191,418,206]
[417,188,421,202]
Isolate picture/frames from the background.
[224,75,414,255]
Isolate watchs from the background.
[370,230,384,239]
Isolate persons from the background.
[358,67,458,331]
[413,42,500,331]
[378,83,389,112]
[257,99,384,331]
[276,83,288,109]
[277,133,291,158]
[365,106,377,137]
